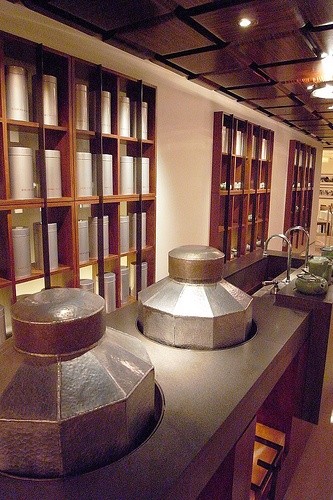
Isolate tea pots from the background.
[295,272,329,295]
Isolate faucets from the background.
[284,226,310,268]
[263,233,292,283]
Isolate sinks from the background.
[223,248,305,296]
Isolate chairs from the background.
[318,219,330,236]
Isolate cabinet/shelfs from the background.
[283,140,317,253]
[209,111,274,265]
[0,29,157,339]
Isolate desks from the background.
[319,210,333,235]
